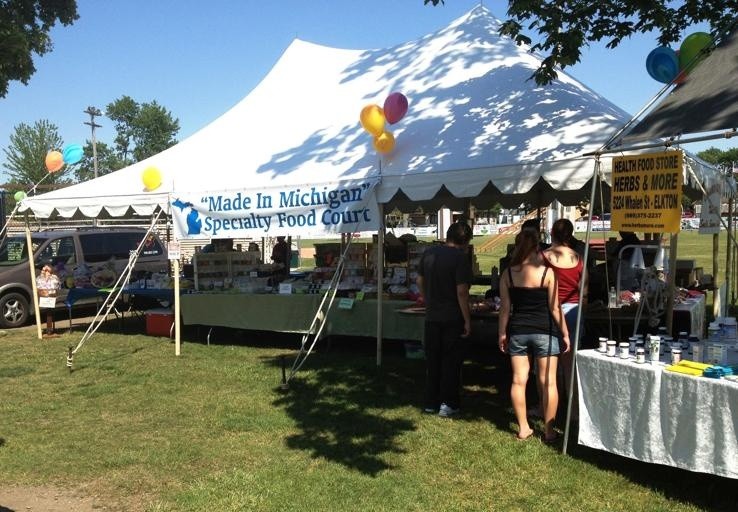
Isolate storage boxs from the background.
[143,305,175,339]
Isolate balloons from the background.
[63,143,84,164]
[143,166,161,190]
[360,103,388,135]
[672,48,688,83]
[679,31,716,72]
[383,92,408,125]
[14,191,25,202]
[373,130,395,154]
[45,150,65,172]
[646,45,681,84]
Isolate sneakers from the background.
[439,403,458,416]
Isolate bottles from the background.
[705,316,738,367]
[636,335,643,341]
[636,349,645,363]
[635,341,645,356]
[645,325,704,367]
[607,286,616,309]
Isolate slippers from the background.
[545,430,562,443]
[516,428,533,441]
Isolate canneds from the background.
[671,349,682,364]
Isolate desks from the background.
[68,271,217,344]
[177,279,497,364]
[572,318,736,484]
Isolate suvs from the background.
[0,225,171,328]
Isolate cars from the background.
[575,213,611,223]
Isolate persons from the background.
[416,220,474,419]
[495,216,643,303]
[35,263,61,336]
[270,235,292,263]
[495,230,572,444]
[534,218,588,427]
